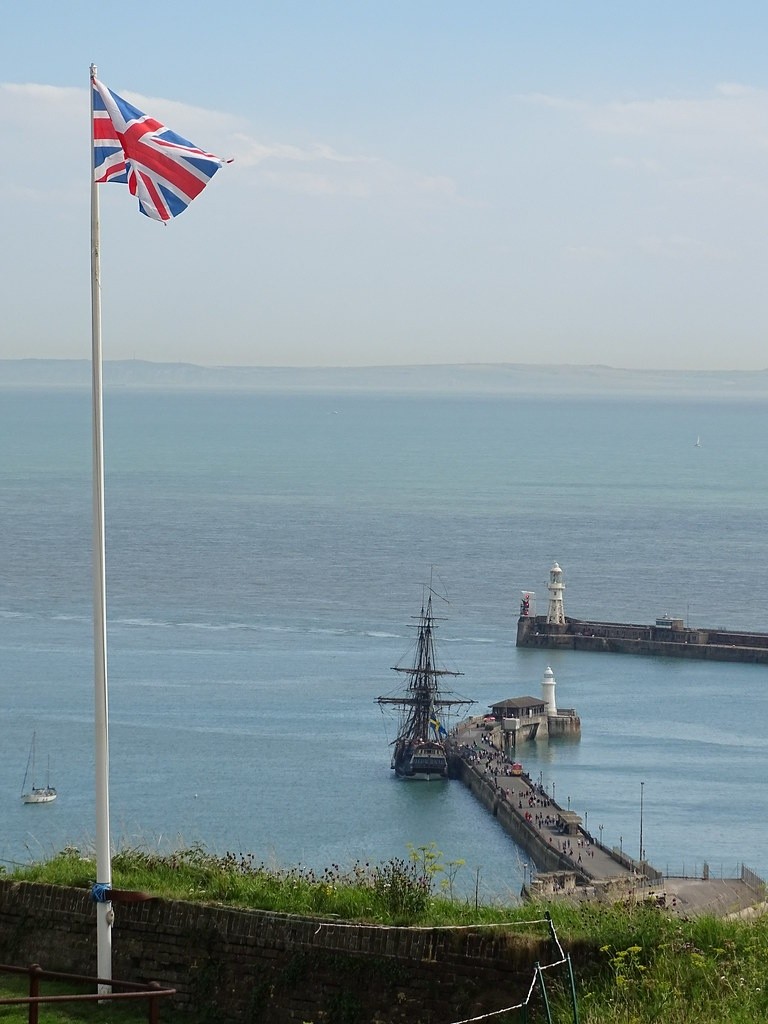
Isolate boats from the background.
[373,629,478,706]
[19,730,58,803]
[390,596,466,676]
[410,566,449,620]
[404,586,439,629]
[390,600,450,781]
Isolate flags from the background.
[92,77,235,228]
[429,715,448,738]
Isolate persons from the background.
[455,717,597,864]
